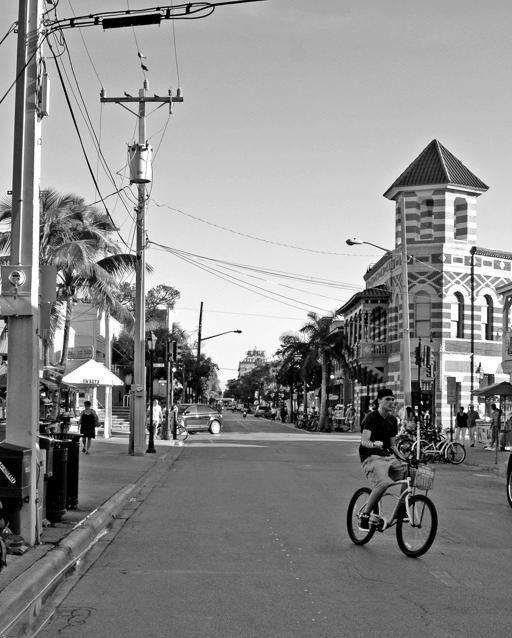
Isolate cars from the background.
[163,403,224,434]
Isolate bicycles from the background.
[395,424,467,466]
[346,456,439,558]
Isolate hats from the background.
[372,388,394,405]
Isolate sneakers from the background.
[357,510,369,531]
[402,514,410,522]
[82,448,90,454]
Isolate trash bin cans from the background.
[39,432,84,523]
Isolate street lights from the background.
[192,329,242,402]
[345,237,394,303]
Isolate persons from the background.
[146,399,164,440]
[357,388,417,532]
[170,400,181,425]
[43,416,65,438]
[485,403,507,451]
[76,401,98,455]
[466,403,480,448]
[454,406,468,446]
[344,403,357,432]
